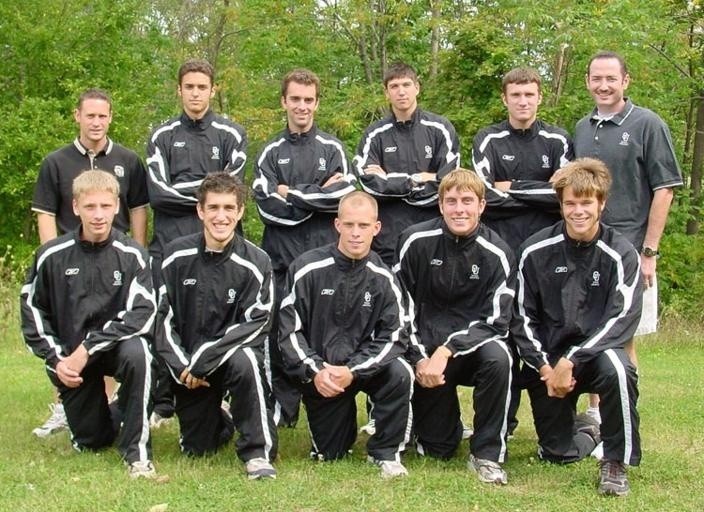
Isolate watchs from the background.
[410,171,424,186]
[637,245,662,259]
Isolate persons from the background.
[25,88,153,443]
[19,167,158,485]
[470,68,584,443]
[274,189,417,482]
[349,60,473,441]
[504,155,645,499]
[248,67,357,429]
[146,170,282,482]
[138,59,246,433]
[388,166,519,485]
[568,49,686,418]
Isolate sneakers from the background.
[123,459,156,481]
[359,419,376,436]
[467,454,507,485]
[148,412,175,430]
[462,424,473,440]
[32,402,69,440]
[221,400,236,431]
[367,455,409,481]
[246,457,277,481]
[586,408,604,460]
[596,456,630,496]
